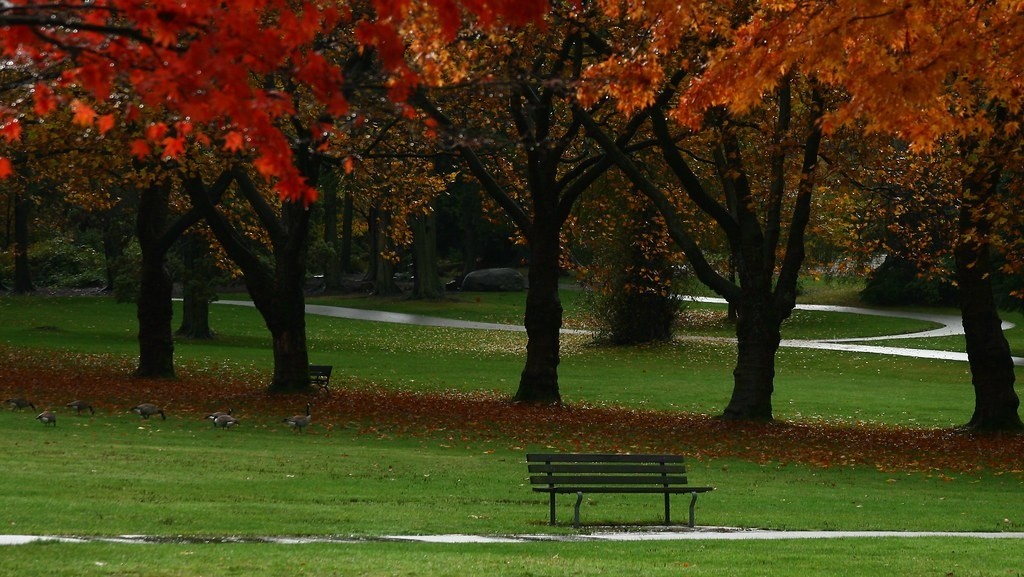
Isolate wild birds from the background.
[129,403,166,420]
[66,400,95,416]
[281,401,313,433]
[36,407,57,426]
[6,399,36,412]
[204,406,239,430]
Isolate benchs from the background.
[525,452,714,527]
[306,364,334,392]
